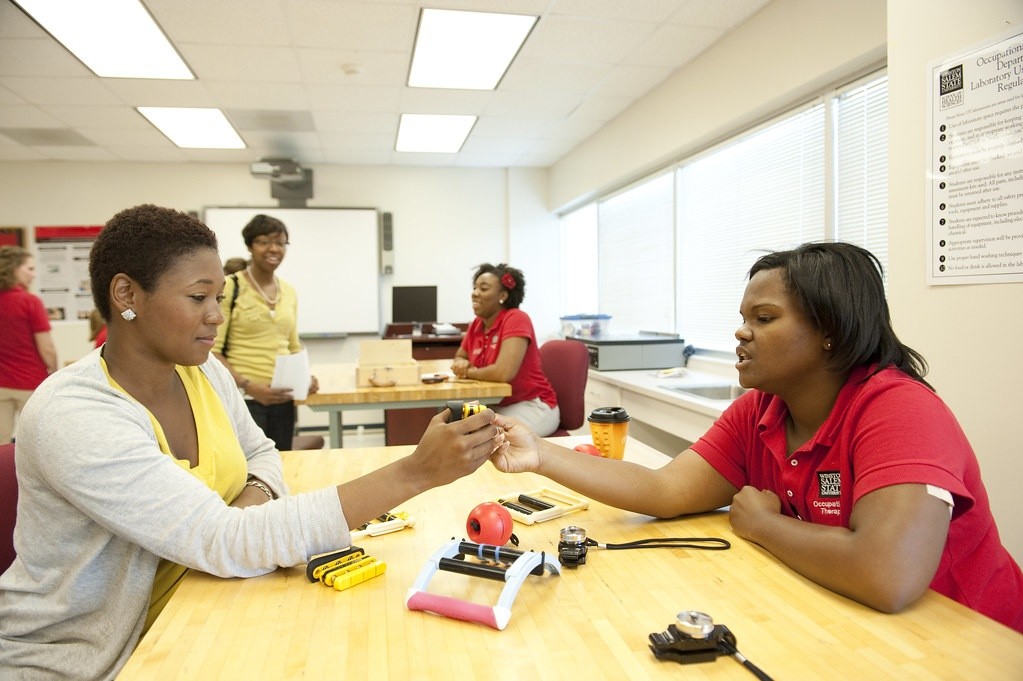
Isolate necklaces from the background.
[248,266,279,304]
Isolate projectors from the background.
[249,162,299,175]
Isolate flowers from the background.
[502,274,517,288]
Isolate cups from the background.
[587,407,631,462]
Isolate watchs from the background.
[238,380,250,395]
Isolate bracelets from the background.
[247,481,272,501]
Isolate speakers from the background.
[380,211,395,274]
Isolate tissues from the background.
[353,339,422,388]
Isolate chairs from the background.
[537,338,592,438]
[285,432,322,450]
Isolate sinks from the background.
[670,384,744,401]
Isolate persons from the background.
[451,266,560,437]
[487,243,1023,633]
[90,311,109,350]
[224,258,247,275]
[0,205,497,681]
[210,215,319,452]
[0,246,58,445]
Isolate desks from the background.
[108,432,1023,681]
[383,323,471,445]
[306,377,513,447]
